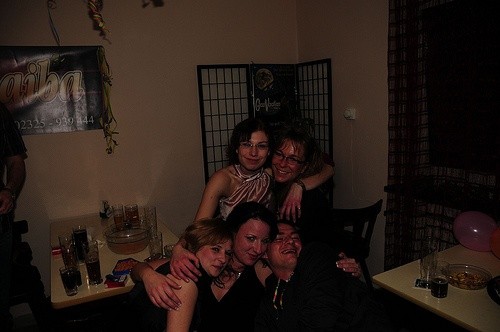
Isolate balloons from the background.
[453,212,500,257]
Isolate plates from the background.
[444,263,492,290]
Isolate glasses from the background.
[272,150,305,166]
[238,141,271,151]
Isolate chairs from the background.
[8,221,47,332]
[332,199,383,290]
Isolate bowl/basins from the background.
[104,223,148,255]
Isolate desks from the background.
[370,244,500,332]
[51,209,179,310]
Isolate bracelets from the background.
[296,179,306,190]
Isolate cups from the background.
[144,207,157,231]
[419,235,437,279]
[164,242,175,256]
[124,203,139,222]
[111,202,124,226]
[72,224,89,261]
[57,231,82,286]
[429,259,449,298]
[83,240,102,284]
[149,232,163,257]
[59,265,77,296]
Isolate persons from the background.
[269,122,334,245]
[0,101,28,332]
[111,218,234,332]
[129,201,368,332]
[170,220,393,332]
[191,117,335,226]
